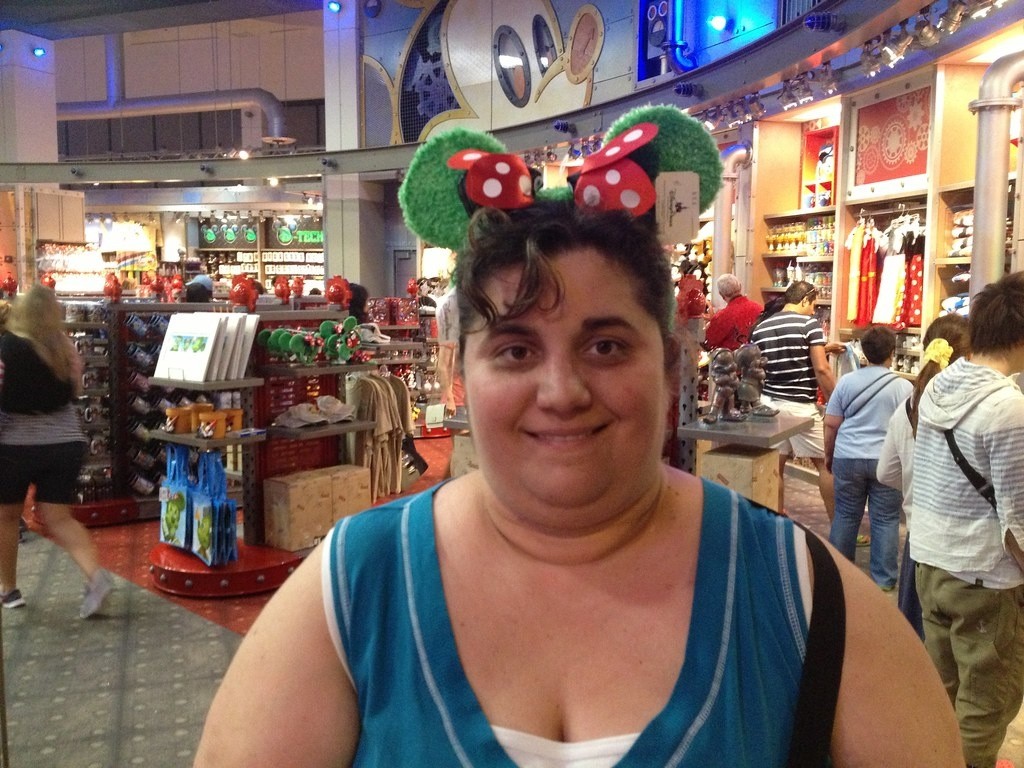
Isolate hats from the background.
[185,274,212,291]
[358,322,391,343]
[275,395,355,429]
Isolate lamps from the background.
[199,165,213,173]
[673,82,704,98]
[553,120,576,133]
[702,0,1011,130]
[319,157,337,168]
[71,168,85,175]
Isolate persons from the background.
[194,105,1024,768]
[0,284,119,618]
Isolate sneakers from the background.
[80,569,117,618]
[0,589,25,608]
[855,533,870,547]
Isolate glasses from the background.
[806,285,816,294]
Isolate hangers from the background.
[858,209,875,225]
[892,204,920,222]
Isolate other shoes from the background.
[995,760,1015,768]
[880,585,895,591]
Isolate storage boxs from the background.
[264,470,332,551]
[318,464,371,525]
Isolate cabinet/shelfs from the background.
[53,293,470,597]
[843,190,930,379]
[933,176,1018,321]
[761,206,836,345]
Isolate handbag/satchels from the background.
[159,442,195,550]
[426,404,449,428]
[192,448,238,567]
